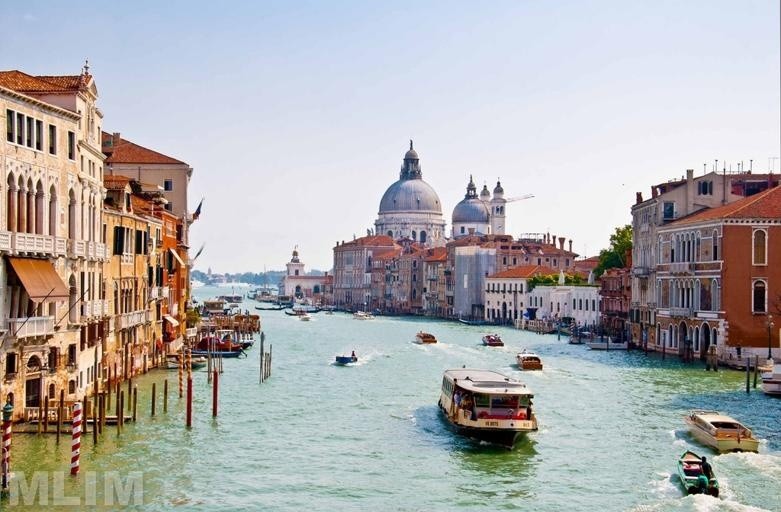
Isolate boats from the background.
[185,294,261,357]
[516,349,542,371]
[761,364,781,396]
[459,304,508,326]
[438,365,538,451]
[676,451,719,498]
[560,317,628,350]
[336,351,357,364]
[685,409,760,453]
[481,334,504,347]
[415,330,437,344]
[246,289,376,320]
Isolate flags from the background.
[194,199,204,220]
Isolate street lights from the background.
[763,315,775,360]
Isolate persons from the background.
[489,336,495,341]
[454,388,534,424]
[417,330,424,337]
[523,348,527,353]
[352,350,356,356]
[495,333,499,341]
[700,456,712,480]
